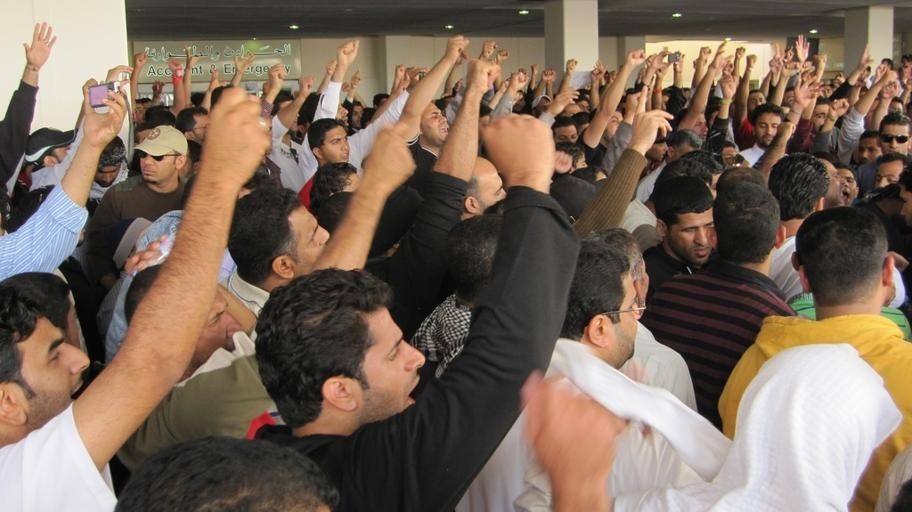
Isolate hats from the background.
[25,128,76,162]
[98,136,126,165]
[132,125,188,156]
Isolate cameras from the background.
[668,52,681,63]
[88,81,119,108]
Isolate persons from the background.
[1,22,912,510]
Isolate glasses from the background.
[881,134,909,143]
[137,148,179,161]
[602,296,646,315]
